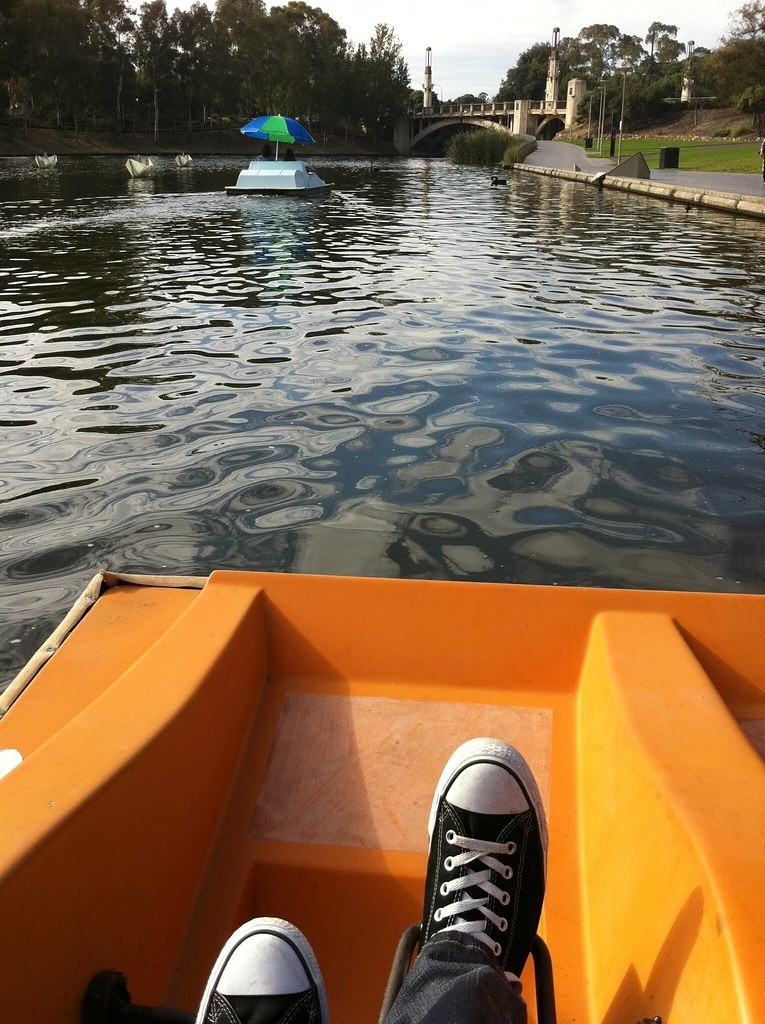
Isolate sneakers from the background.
[195,916,330,1024]
[417,736,550,984]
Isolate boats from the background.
[175,151,192,167]
[35,151,57,169]
[125,153,155,178]
[224,160,335,199]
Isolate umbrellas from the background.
[240,114,316,161]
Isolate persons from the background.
[760,139,765,184]
[255,145,275,161]
[283,149,315,171]
[196,736,549,1024]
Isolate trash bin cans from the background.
[584,138,593,148]
[659,146,680,170]
